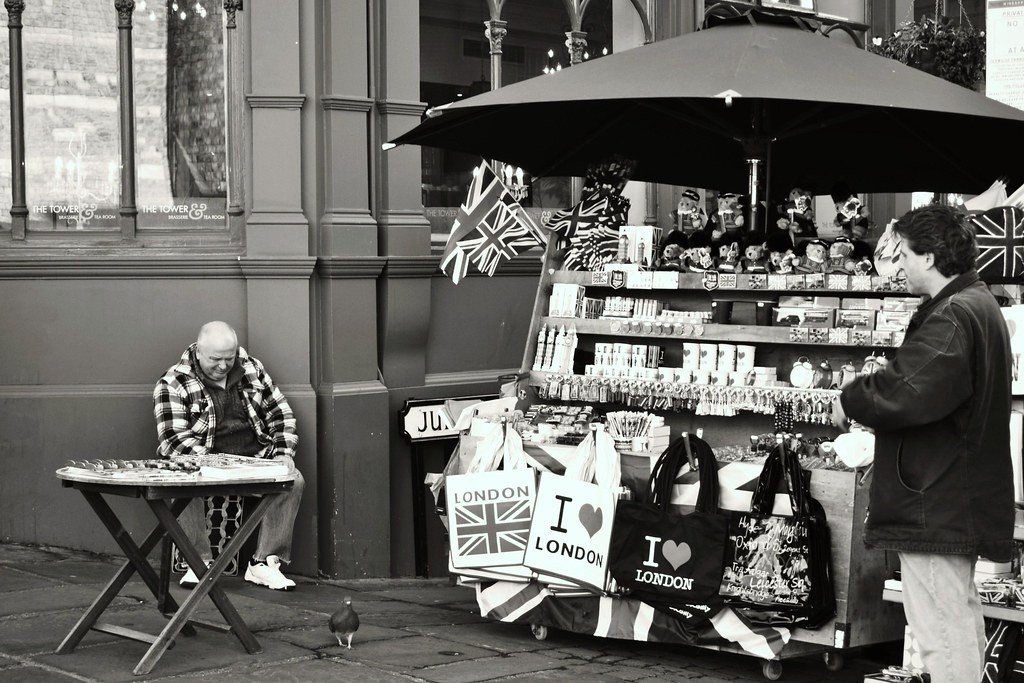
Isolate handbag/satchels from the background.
[431,425,837,660]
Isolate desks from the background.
[55,458,298,675]
[880,588,1024,622]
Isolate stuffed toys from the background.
[657,184,876,277]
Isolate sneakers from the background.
[179,565,212,589]
[244,555,296,592]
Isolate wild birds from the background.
[328,596,360,651]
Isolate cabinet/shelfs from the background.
[518,228,927,478]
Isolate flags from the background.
[436,161,632,285]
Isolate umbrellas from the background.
[382,22,1024,234]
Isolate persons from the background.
[151,320,306,592]
[830,203,1017,683]
